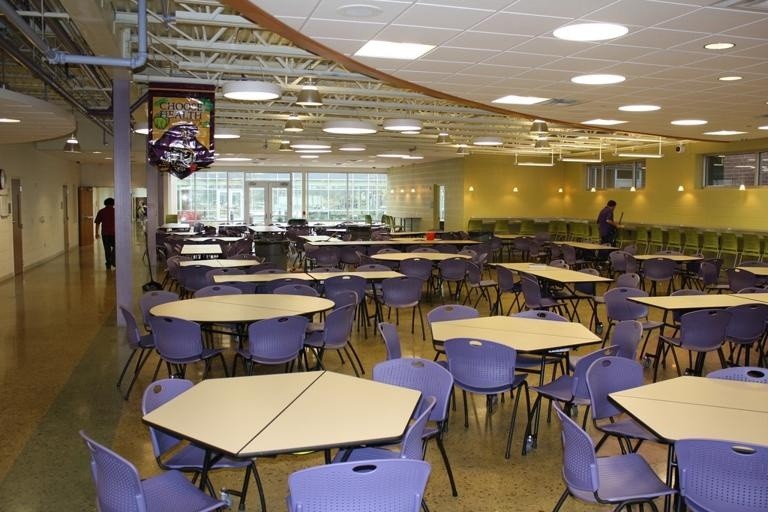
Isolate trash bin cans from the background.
[252,238,291,272]
[142,280,163,291]
[346,224,372,241]
[287,218,309,225]
[467,230,489,243]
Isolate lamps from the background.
[615,136,662,158]
[134,124,148,134]
[63,133,82,153]
[559,137,601,162]
[214,72,550,159]
[514,149,554,167]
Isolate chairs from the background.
[141,378,269,511]
[117,215,768,376]
[549,400,677,510]
[287,457,432,510]
[674,438,768,511]
[585,356,677,457]
[704,366,766,383]
[441,339,535,461]
[340,356,461,500]
[526,345,624,451]
[79,429,227,512]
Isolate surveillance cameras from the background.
[674,143,683,154]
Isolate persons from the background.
[595,200,625,271]
[94,198,115,269]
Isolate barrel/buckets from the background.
[425,230,436,241]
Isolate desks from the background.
[140,370,423,511]
[607,374,768,511]
